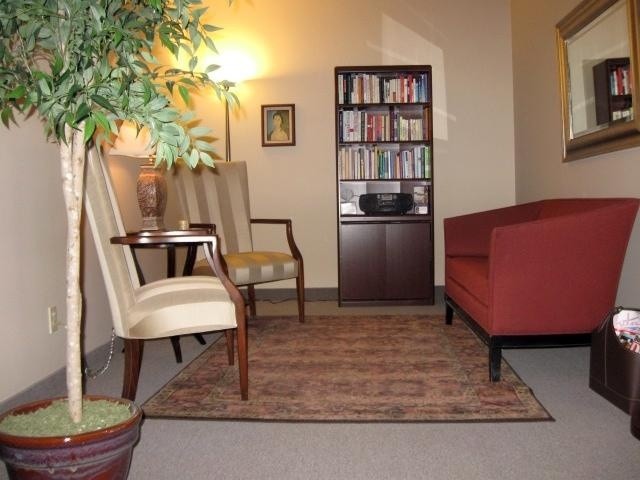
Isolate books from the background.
[335,73,431,181]
[413,185,429,215]
[607,65,634,124]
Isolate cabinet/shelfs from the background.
[592,57,633,125]
[333,65,435,307]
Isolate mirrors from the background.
[554,0,640,163]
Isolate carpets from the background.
[139,315,555,422]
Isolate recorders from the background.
[359,193,413,216]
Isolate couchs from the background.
[444,196,639,381]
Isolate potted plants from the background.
[0,0,246,480]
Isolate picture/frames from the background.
[260,104,297,148]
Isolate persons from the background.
[267,111,290,142]
